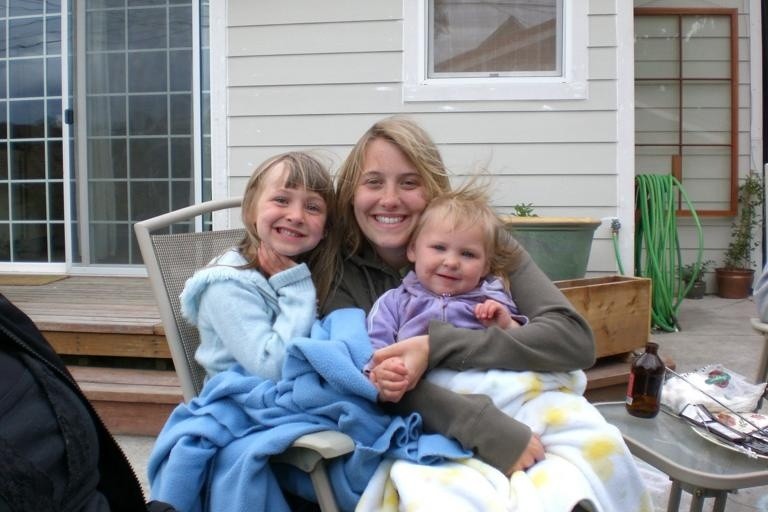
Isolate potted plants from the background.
[494,201,602,280]
[715,169,764,299]
[677,259,714,299]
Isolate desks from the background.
[593,401,768,512]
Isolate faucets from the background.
[610,217,621,235]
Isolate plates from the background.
[690,410,768,459]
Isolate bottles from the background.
[624,341,666,418]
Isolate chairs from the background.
[134,195,356,511]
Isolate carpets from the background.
[0,274,72,286]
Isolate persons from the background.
[177,149,345,399]
[299,113,598,480]
[363,191,532,404]
